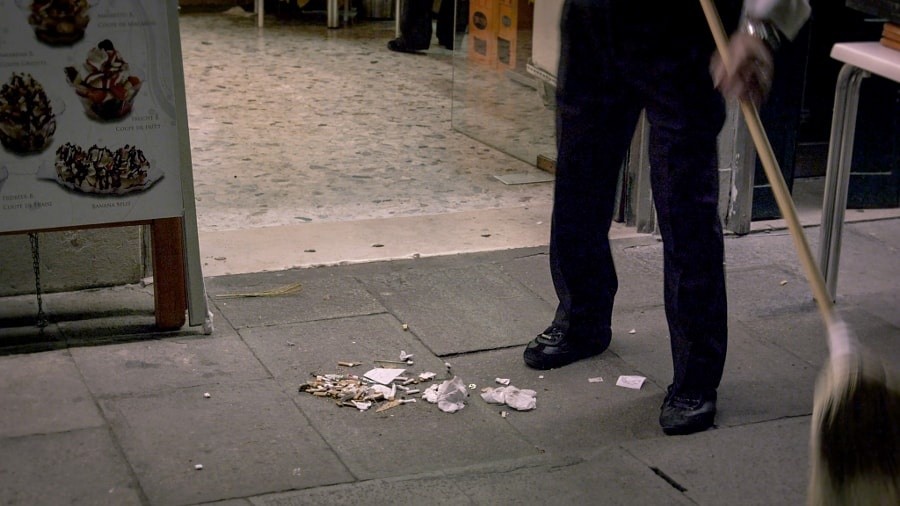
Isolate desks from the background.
[816,41,900,302]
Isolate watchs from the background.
[734,16,782,51]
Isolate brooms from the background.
[699,0,900,506]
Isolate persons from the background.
[523,0,813,436]
[386,0,469,52]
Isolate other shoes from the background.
[388,37,429,50]
[438,39,461,50]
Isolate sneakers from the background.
[522,325,613,371]
[658,384,717,435]
